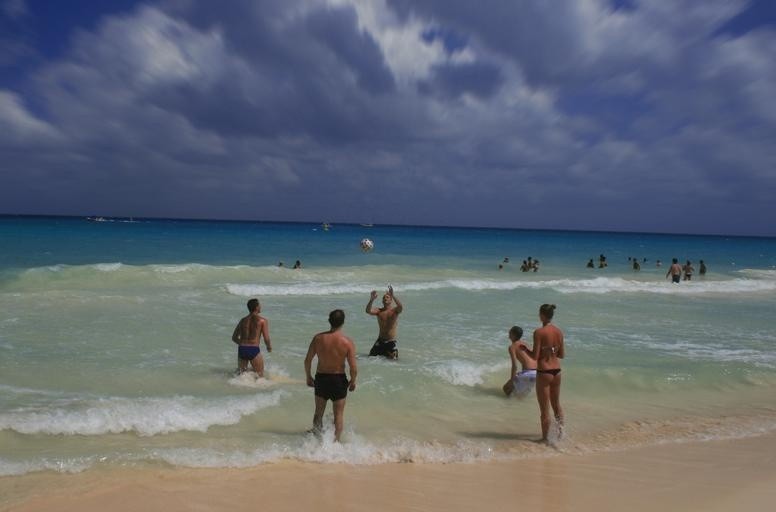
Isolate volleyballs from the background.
[360,238,374,250]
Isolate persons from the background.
[684,259,696,281]
[586,253,661,271]
[519,303,565,444]
[666,257,682,283]
[293,260,302,269]
[699,259,706,276]
[231,297,271,379]
[503,326,538,400]
[303,309,357,444]
[366,284,403,360]
[497,255,546,273]
